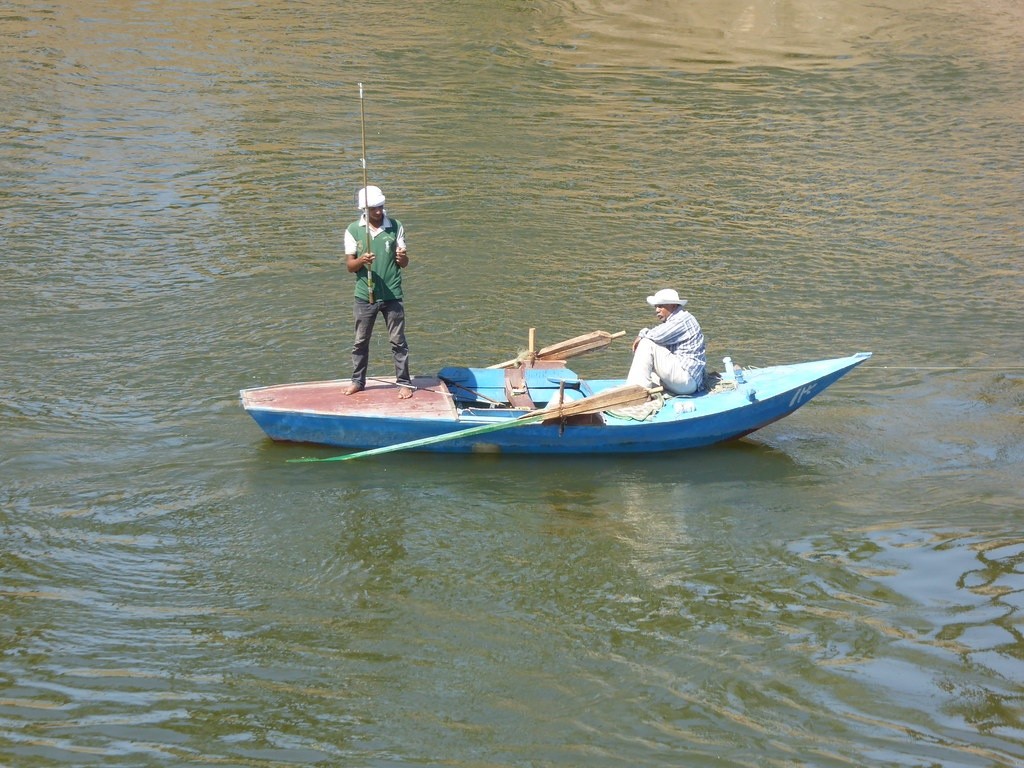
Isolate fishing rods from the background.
[358,80,375,304]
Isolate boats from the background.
[237,326,874,466]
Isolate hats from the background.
[647,288,687,306]
[358,186,385,210]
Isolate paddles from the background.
[486,329,627,372]
[285,382,665,465]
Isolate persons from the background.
[623,288,707,397]
[344,186,414,401]
